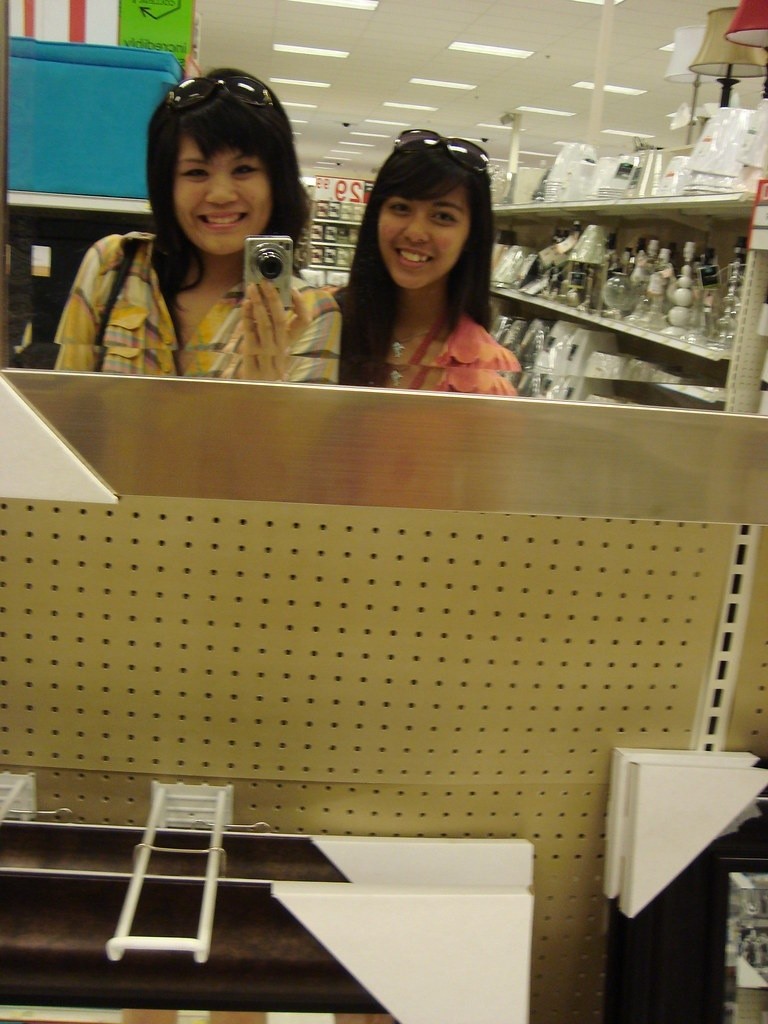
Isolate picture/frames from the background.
[0,820,535,888]
[604,746,768,1023]
[622,761,767,1024]
[709,848,767,1024]
[0,866,534,1024]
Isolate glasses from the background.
[395,128,490,171]
[165,77,274,113]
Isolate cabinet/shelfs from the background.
[491,179,768,416]
[309,218,362,271]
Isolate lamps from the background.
[567,222,608,311]
[690,7,768,108]
[662,0,718,145]
[724,0,768,99]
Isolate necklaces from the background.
[387,365,413,386]
[387,317,438,358]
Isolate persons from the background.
[334,356,521,398]
[309,129,522,372]
[48,67,342,360]
[53,341,340,388]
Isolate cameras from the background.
[245,235,295,312]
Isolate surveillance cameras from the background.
[337,163,341,165]
[344,123,349,127]
[482,138,489,143]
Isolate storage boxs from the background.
[7,36,185,201]
[7,1,119,48]
[119,0,194,67]
